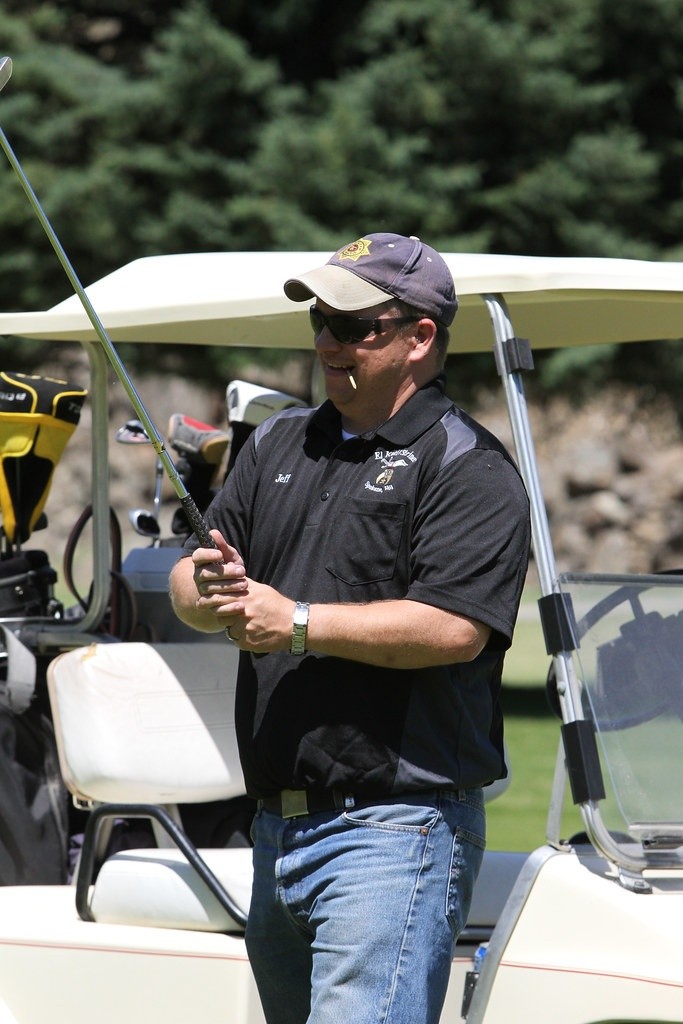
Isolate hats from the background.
[283,233,459,326]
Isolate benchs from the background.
[45,640,532,940]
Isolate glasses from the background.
[310,303,429,344]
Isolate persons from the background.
[165,232,535,1022]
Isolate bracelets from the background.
[291,599,306,658]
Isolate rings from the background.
[224,625,236,644]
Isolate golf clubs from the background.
[0,57,271,659]
[113,416,164,548]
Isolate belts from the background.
[261,787,391,818]
